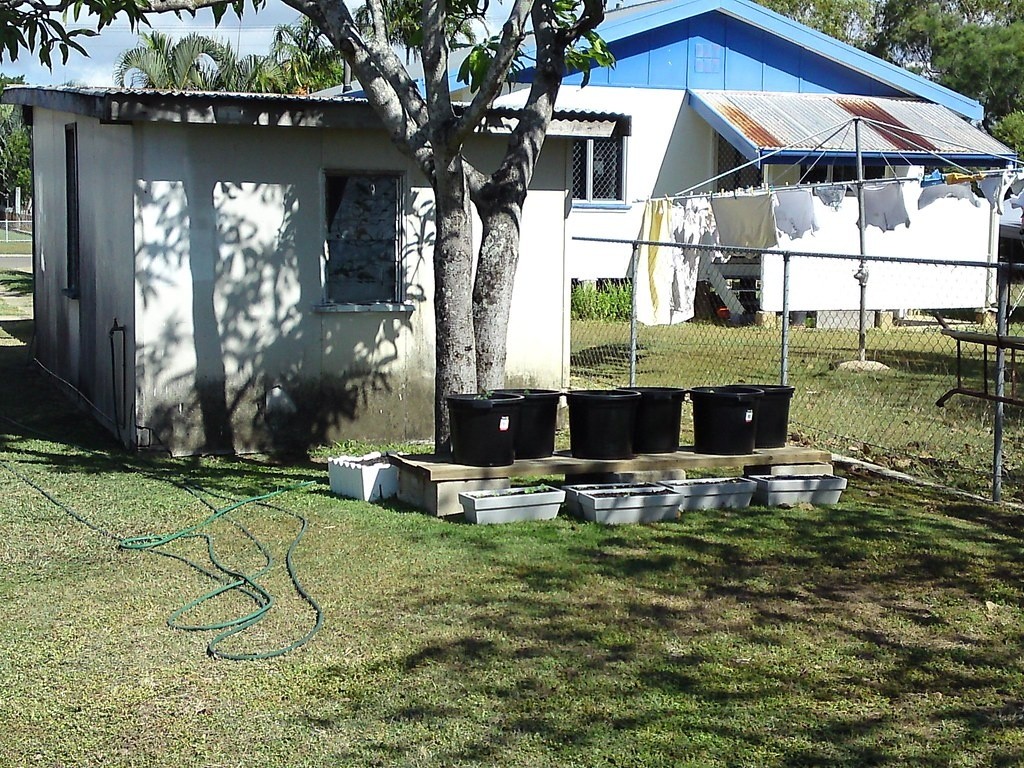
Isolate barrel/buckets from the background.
[446,384,795,467]
